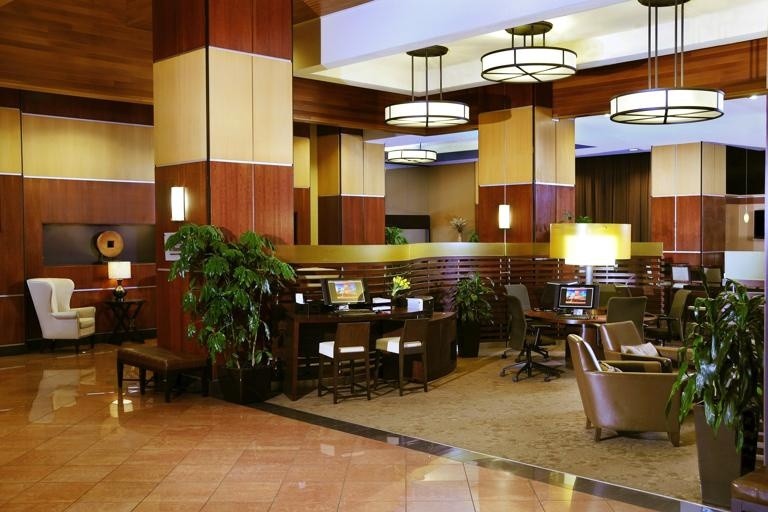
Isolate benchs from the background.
[117,346,210,403]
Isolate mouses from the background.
[375,309,381,314]
[593,315,598,320]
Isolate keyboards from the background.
[333,309,375,318]
[560,314,591,320]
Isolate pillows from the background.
[621,342,660,357]
[600,362,622,373]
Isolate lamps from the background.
[744,149,750,223]
[388,143,436,164]
[497,202,510,230]
[108,261,131,302]
[385,45,470,129]
[549,222,632,285]
[610,0,724,126]
[481,21,576,83]
[170,186,186,223]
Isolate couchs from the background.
[567,333,682,446]
[599,320,686,373]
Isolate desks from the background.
[272,295,433,401]
[525,310,658,352]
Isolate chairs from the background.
[608,297,649,343]
[595,283,619,307]
[500,292,551,383]
[504,284,544,337]
[646,288,694,346]
[27,277,96,354]
[615,287,632,298]
[374,318,430,396]
[318,323,371,403]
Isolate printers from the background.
[393,295,434,314]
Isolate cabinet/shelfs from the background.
[378,312,457,382]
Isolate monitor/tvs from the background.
[538,281,579,312]
[669,265,691,283]
[321,276,370,310]
[554,284,600,315]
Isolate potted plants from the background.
[163,223,297,404]
[664,277,766,508]
[448,272,497,358]
